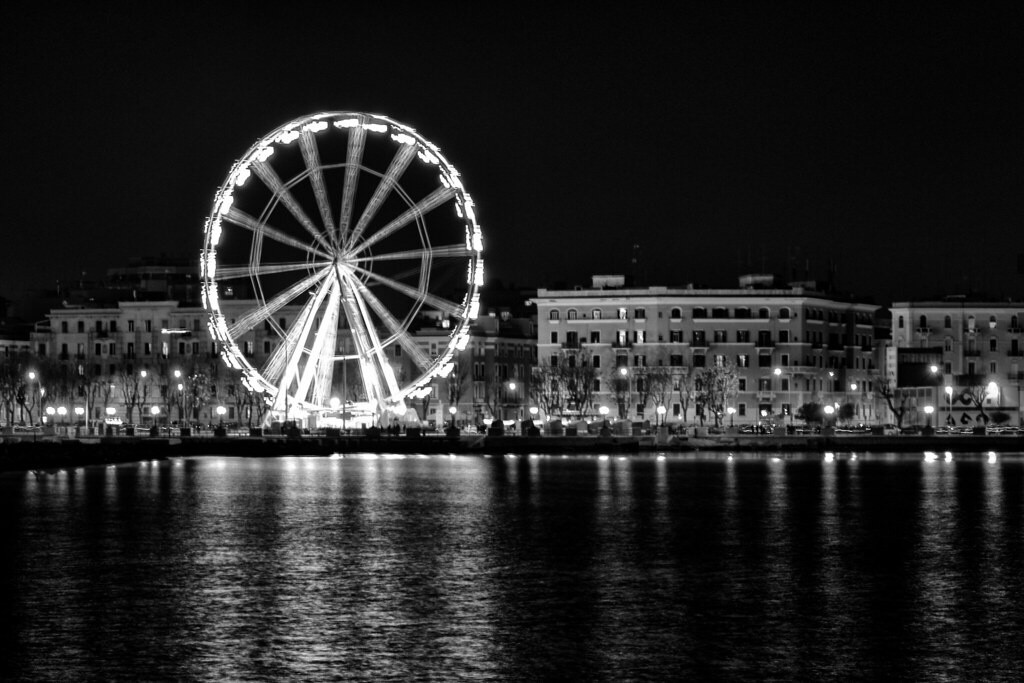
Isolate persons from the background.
[74,423,80,438]
[751,424,771,434]
[388,423,406,437]
[818,426,821,434]
[191,422,200,434]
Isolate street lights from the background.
[930,365,953,427]
[621,368,632,424]
[149,405,161,426]
[175,370,186,428]
[29,373,43,429]
[216,406,227,429]
[449,406,458,427]
